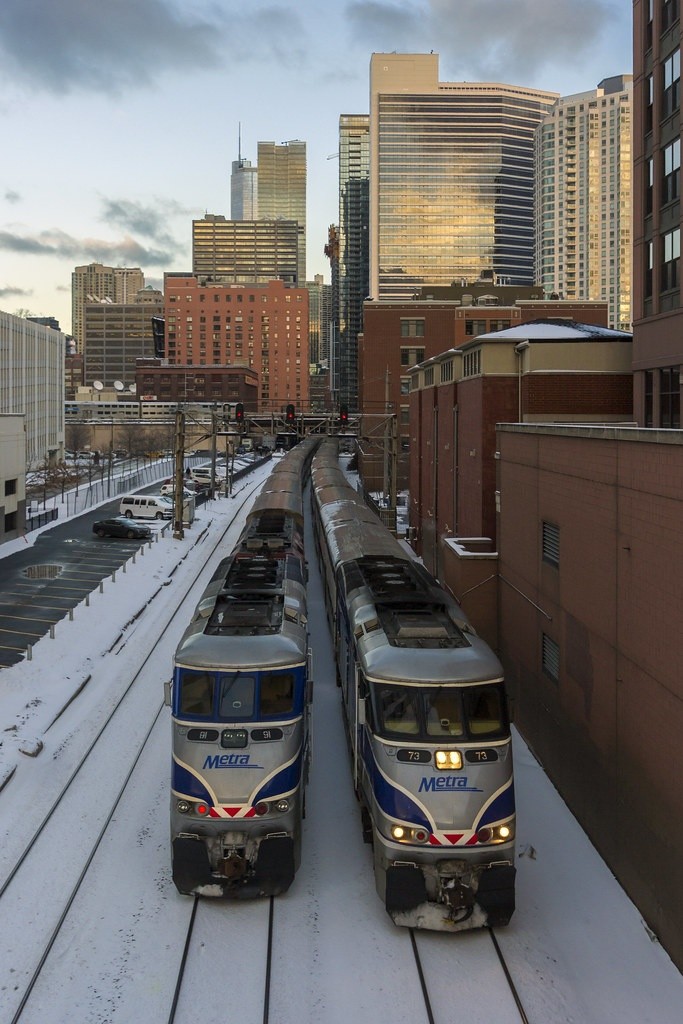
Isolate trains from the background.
[276,435,305,450]
[160,431,519,932]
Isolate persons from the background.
[185,466,191,479]
[193,479,200,494]
[473,689,491,722]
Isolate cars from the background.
[157,496,175,504]
[65,448,127,460]
[93,516,152,539]
[25,468,89,487]
[235,446,245,454]
[166,491,191,499]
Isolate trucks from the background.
[242,438,254,452]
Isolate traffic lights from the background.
[340,406,348,424]
[286,404,294,421]
[237,404,243,423]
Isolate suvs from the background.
[160,484,197,495]
[192,474,217,484]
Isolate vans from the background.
[119,494,175,520]
[191,468,216,477]
